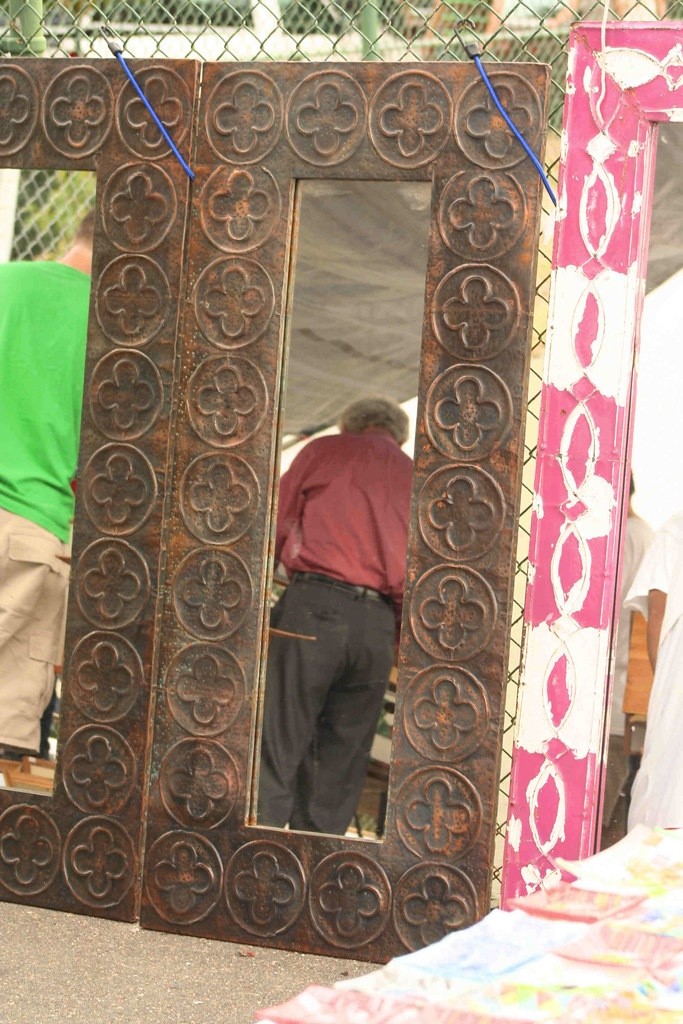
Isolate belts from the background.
[293,570,392,605]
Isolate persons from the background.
[620,507,680,834]
[254,397,415,834]
[1,211,97,758]
[600,472,655,839]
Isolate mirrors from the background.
[500,20,681,906]
[2,57,548,966]
